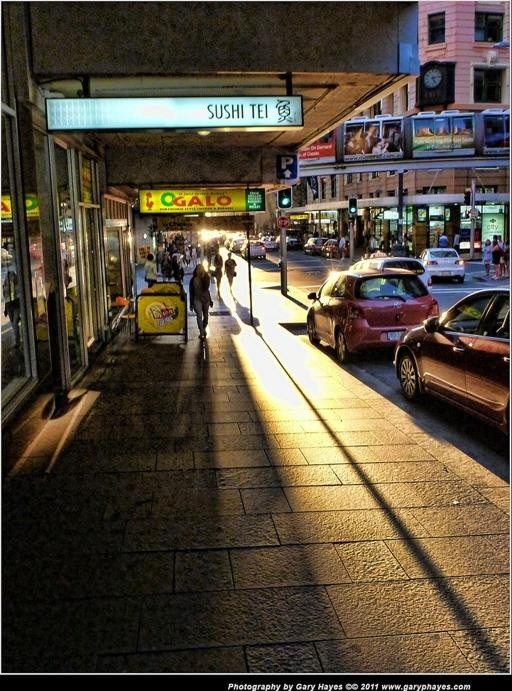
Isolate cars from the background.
[303,238,350,258]
[306,247,510,439]
[224,235,298,259]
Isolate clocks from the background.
[423,67,443,88]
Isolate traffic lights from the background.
[349,199,357,214]
[278,189,291,209]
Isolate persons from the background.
[438,233,448,248]
[338,233,345,261]
[453,230,460,253]
[141,239,237,294]
[484,235,509,282]
[188,264,213,339]
[368,232,412,251]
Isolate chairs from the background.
[115,287,155,337]
